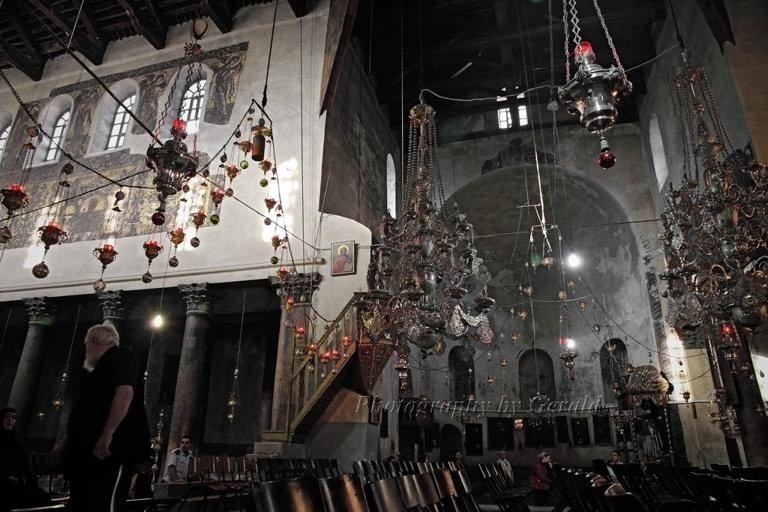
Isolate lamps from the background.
[50,303,89,408]
[144,41,204,226]
[222,289,254,420]
[553,2,631,168]
[629,67,765,370]
[0,98,349,381]
[555,296,581,380]
[353,103,498,353]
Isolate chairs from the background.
[128,449,766,512]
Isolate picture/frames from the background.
[330,240,355,277]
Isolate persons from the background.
[496,450,513,482]
[609,451,623,464]
[83,325,151,511]
[0,406,50,511]
[453,450,463,464]
[538,452,552,469]
[163,435,195,481]
